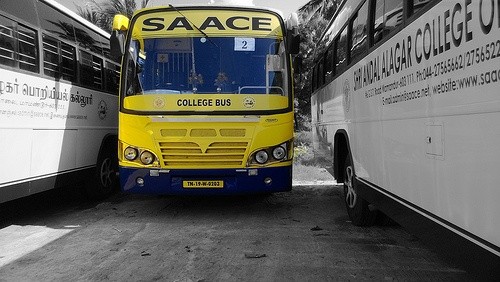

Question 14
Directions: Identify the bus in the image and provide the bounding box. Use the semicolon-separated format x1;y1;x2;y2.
0;0;146;204
109;3;301;195
309;0;499;282
